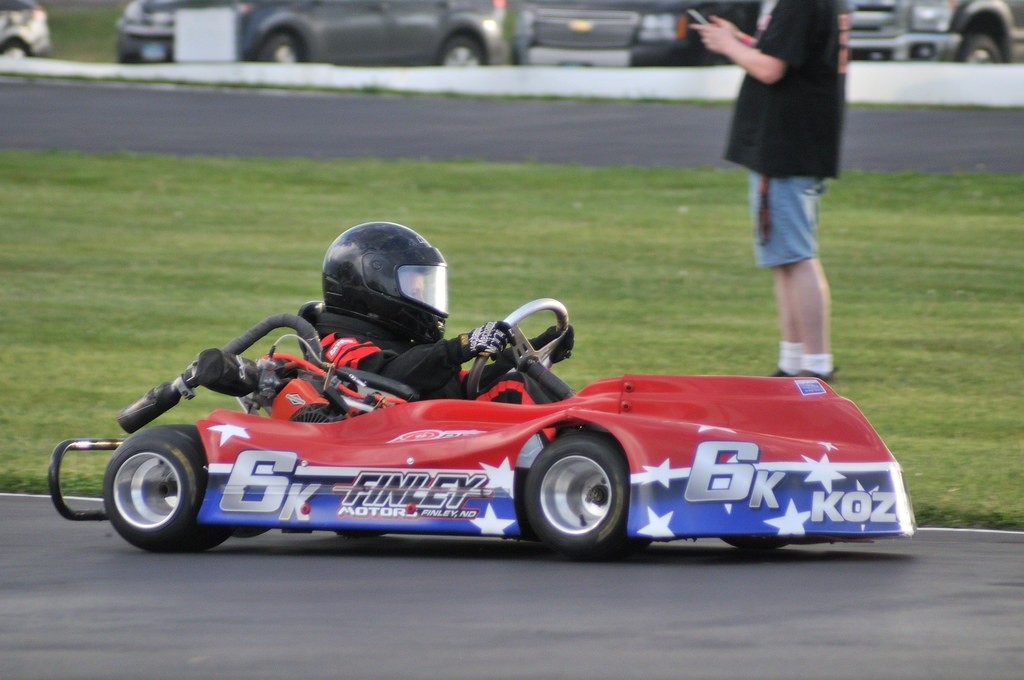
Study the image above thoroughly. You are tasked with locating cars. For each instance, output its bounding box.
[0,1,52,59]
[116,1,1023,66]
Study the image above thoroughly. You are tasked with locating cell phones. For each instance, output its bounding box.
[686,8,709,25]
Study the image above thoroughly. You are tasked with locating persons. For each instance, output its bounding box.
[688,0,850,383]
[313,222,575,405]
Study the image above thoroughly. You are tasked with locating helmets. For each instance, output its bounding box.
[322,221,449,343]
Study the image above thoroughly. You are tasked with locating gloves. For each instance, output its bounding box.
[528,324,574,363]
[458,320,516,363]
